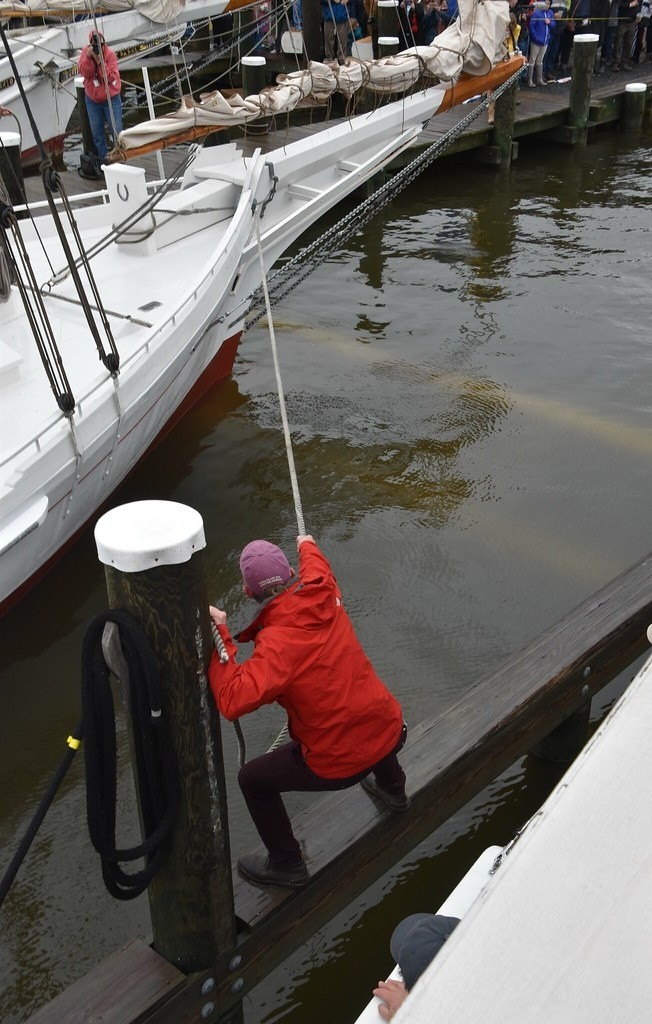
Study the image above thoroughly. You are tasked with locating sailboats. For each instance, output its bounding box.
[0,0,531,627]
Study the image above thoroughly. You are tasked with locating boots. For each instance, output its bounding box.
[528,65,537,87]
[535,62,547,86]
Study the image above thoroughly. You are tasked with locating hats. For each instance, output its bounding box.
[89,31,105,46]
[240,539,291,598]
[389,912,462,989]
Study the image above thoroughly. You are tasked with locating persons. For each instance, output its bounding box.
[372,912,462,1023]
[209,534,410,888]
[293,1,652,124]
[78,31,123,161]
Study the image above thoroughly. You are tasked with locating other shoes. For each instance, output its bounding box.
[362,772,410,812]
[612,61,620,72]
[238,850,312,889]
[623,62,632,70]
[599,59,606,72]
